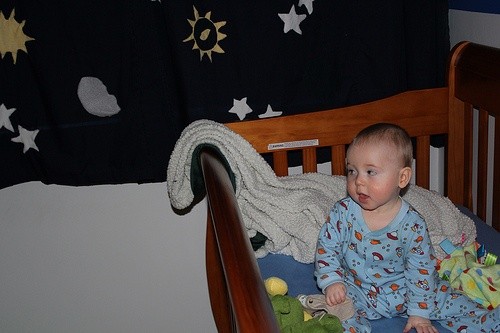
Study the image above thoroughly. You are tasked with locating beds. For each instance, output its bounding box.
[200,41,500,333]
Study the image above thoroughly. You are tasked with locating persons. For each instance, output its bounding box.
[313,123,500,333]
